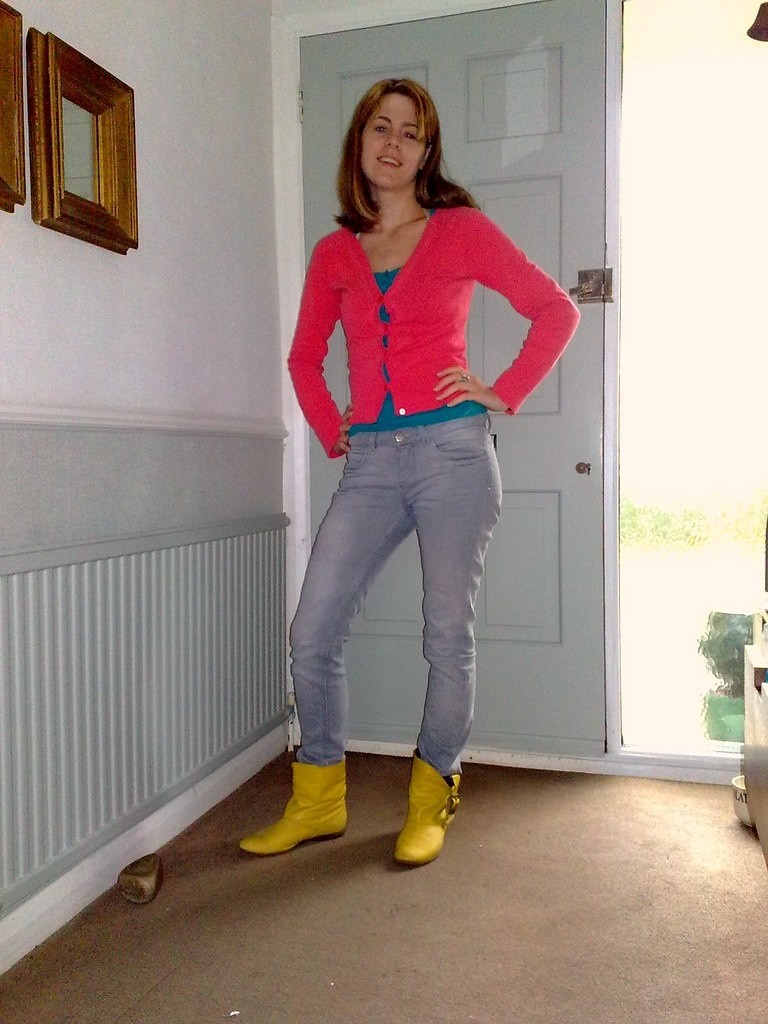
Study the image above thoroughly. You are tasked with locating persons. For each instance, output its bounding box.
[237,78,579,865]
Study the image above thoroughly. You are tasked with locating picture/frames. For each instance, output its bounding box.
[0,1,138,254]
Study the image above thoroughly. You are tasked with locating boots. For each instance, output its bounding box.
[394,748,461,865]
[240,755,348,856]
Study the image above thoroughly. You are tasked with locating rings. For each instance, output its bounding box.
[462,374,470,382]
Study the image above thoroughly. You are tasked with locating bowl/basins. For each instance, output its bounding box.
[732,776,755,827]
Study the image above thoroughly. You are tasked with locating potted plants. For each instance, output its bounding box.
[697,612,753,740]
